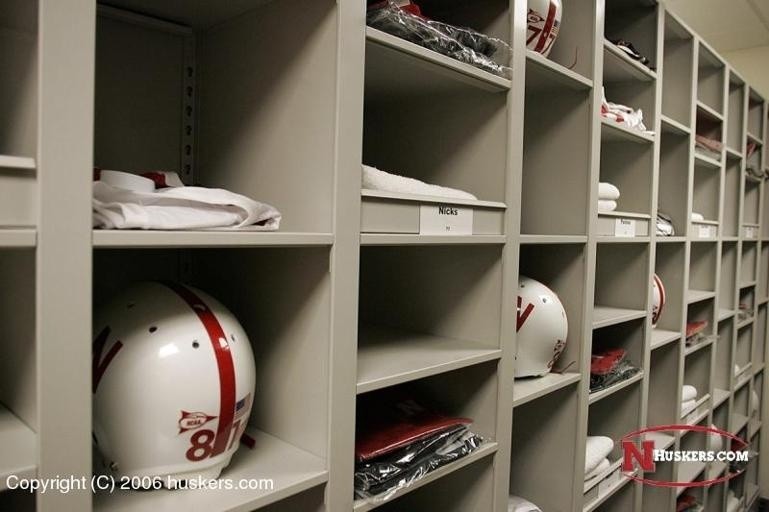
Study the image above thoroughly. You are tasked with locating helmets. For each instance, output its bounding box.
[92,278,256,489]
[527,0,562,59]
[515,276,569,380]
[653,273,666,329]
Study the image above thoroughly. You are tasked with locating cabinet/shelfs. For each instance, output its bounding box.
[0,0,769,512]
